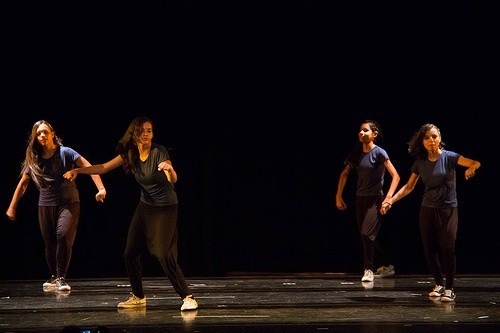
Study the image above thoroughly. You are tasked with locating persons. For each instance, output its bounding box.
[336,120,400,282]
[380,123,481,301]
[6,120,106,290]
[63,117,198,311]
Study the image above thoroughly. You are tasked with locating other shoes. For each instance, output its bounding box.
[42,274,57,287]
[361,268,374,282]
[440,287,456,302]
[373,265,395,278]
[428,284,446,297]
[55,276,72,290]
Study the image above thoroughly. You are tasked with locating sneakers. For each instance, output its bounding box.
[180,295,199,311]
[116,291,146,309]
